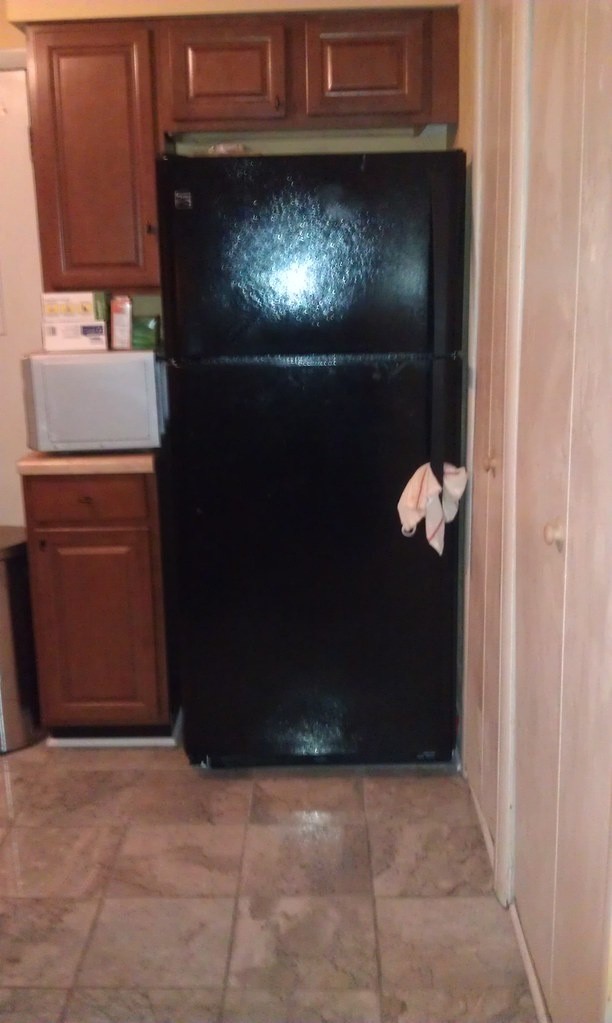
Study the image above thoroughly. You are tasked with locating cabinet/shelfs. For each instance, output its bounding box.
[20,9,459,292]
[25,474,169,731]
